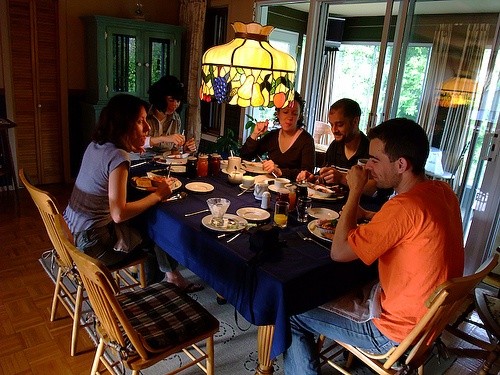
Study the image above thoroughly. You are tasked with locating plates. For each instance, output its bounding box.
[202,214,248,232]
[307,219,339,243]
[221,167,246,174]
[236,207,270,221]
[308,207,339,220]
[135,177,182,191]
[156,158,187,165]
[185,182,214,192]
[307,181,345,201]
[239,184,254,192]
[268,184,297,194]
[240,163,271,174]
[166,165,186,173]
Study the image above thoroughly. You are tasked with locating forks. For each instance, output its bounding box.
[296,231,331,252]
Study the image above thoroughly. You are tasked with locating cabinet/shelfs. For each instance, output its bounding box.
[69,14,184,174]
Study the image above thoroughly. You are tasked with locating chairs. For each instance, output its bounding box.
[18,168,220,375]
[316,246,500,375]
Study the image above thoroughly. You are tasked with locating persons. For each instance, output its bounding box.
[282,117,464,375]
[295,98,395,212]
[225,91,316,181]
[132,75,185,152]
[63,94,202,293]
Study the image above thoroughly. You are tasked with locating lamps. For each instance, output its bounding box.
[438,72,477,107]
[199,0,297,109]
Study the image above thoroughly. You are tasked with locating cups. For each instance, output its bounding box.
[296,196,312,223]
[226,157,241,173]
[275,177,290,191]
[206,198,230,228]
[274,201,289,228]
[357,159,371,179]
[242,176,254,187]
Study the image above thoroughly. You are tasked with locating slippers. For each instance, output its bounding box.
[182,281,205,293]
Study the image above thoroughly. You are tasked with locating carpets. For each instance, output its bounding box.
[39,258,500,375]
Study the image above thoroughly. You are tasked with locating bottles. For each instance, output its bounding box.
[185,153,222,179]
[260,191,271,209]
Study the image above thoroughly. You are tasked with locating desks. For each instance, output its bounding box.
[126,159,379,375]
